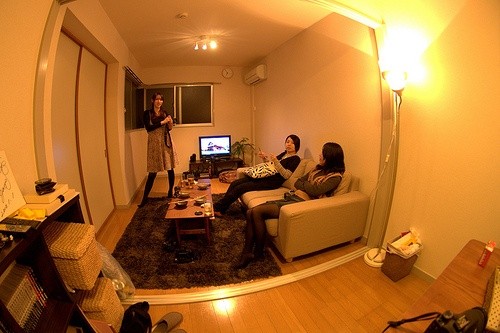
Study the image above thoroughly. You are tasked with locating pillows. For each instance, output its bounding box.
[242,160,280,178]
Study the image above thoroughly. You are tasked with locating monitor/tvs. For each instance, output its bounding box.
[199,135,232,160]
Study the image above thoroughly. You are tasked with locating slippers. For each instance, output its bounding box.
[150,312,182,333]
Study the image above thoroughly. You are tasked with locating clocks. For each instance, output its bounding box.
[222,66,234,79]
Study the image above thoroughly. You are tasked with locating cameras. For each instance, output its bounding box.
[423,306,488,333]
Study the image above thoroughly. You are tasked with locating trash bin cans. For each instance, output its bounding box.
[382,232,417,282]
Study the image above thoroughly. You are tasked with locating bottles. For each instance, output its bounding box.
[479,240,496,268]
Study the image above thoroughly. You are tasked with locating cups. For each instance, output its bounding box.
[204,203,212,215]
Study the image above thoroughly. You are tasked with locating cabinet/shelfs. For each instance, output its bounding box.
[0,191,105,333]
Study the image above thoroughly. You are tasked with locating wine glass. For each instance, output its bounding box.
[179,174,185,188]
[187,175,192,188]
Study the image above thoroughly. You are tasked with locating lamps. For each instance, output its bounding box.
[191,35,220,52]
[364,67,410,268]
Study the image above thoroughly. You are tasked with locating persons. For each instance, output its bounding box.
[231,142,345,269]
[213,135,301,217]
[137,92,177,208]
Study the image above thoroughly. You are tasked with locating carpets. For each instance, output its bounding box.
[110,192,285,291]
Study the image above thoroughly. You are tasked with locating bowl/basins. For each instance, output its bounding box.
[175,200,187,207]
[195,198,206,204]
[198,185,207,189]
[179,192,190,198]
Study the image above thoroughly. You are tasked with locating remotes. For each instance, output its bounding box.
[0,223,32,235]
[1,218,41,230]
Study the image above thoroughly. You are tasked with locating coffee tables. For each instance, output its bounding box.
[165,177,215,245]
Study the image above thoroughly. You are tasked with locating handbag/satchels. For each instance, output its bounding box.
[386,229,423,259]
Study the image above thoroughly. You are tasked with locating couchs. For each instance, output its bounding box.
[235,159,371,264]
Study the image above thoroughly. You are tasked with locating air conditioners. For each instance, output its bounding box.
[244,63,268,88]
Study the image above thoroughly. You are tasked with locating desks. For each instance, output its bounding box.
[387,237,500,333]
[183,156,244,179]
